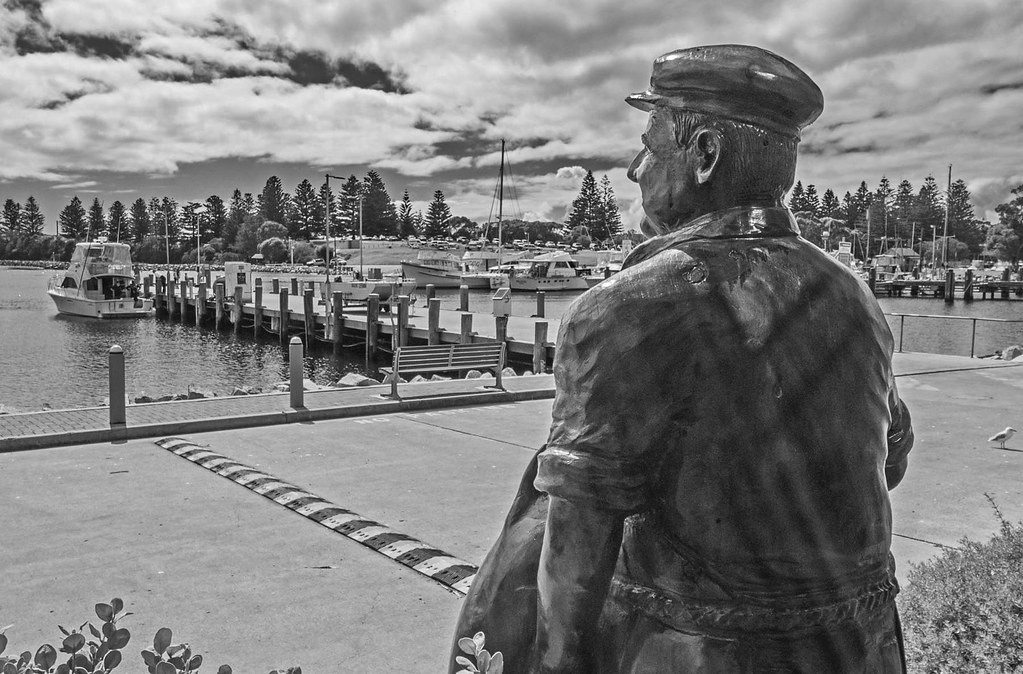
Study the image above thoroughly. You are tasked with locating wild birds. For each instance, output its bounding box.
[988,427,1018,449]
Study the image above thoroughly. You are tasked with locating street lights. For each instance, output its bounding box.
[324,172,347,280]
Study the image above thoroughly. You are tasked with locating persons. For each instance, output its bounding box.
[451,47,913,674]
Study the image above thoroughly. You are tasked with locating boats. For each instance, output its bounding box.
[400,251,463,287]
[512,251,591,292]
[46,240,156,322]
[318,279,412,302]
[463,250,512,290]
